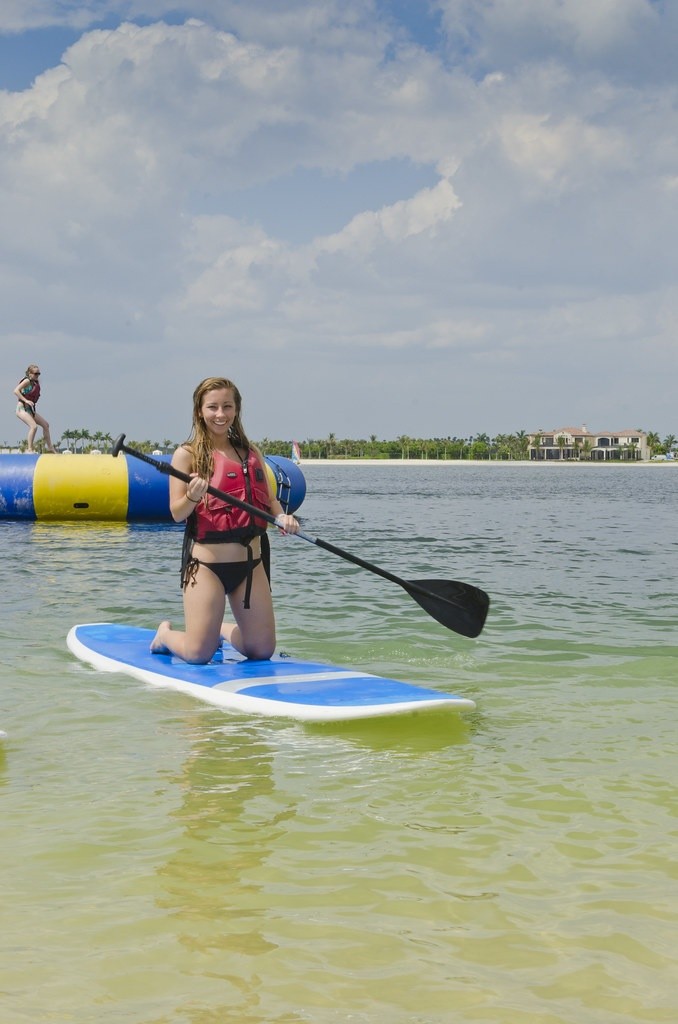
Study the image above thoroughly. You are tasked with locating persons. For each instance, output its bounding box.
[14,365,57,454]
[149,377,300,665]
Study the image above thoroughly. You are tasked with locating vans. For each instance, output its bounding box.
[652,455,666,460]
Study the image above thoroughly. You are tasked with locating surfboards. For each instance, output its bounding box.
[65,621,478,725]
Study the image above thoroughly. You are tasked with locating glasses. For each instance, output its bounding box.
[30,373,41,375]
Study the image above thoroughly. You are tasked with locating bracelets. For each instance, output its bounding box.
[186,494,199,502]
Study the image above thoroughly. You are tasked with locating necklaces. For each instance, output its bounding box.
[230,442,243,462]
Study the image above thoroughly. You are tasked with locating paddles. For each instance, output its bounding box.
[112,433,491,639]
